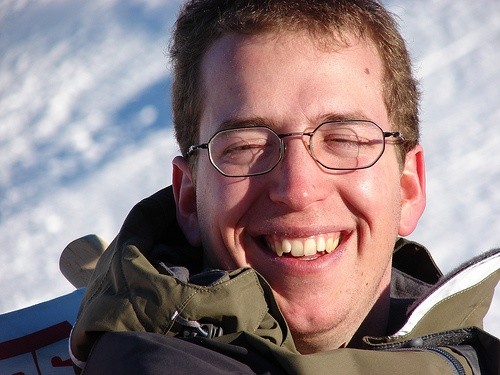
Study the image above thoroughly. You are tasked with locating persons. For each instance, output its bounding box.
[69,0,499,375]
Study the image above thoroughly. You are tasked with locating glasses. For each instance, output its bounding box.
[182,120,406,177]
